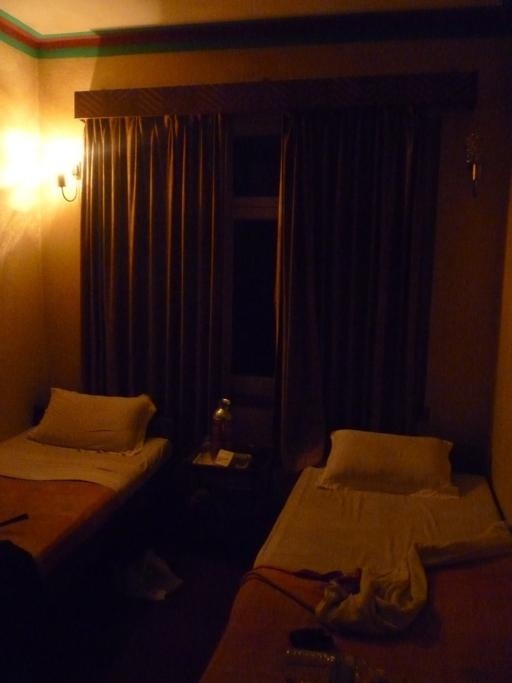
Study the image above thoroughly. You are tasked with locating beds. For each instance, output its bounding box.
[1,429,171,591]
[198,466,512,682]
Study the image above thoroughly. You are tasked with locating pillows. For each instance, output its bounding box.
[315,430,455,500]
[25,387,155,457]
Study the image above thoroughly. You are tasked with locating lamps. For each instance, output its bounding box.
[41,142,82,201]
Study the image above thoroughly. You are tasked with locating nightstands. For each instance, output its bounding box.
[186,446,277,567]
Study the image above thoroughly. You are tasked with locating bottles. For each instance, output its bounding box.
[212,397,235,459]
